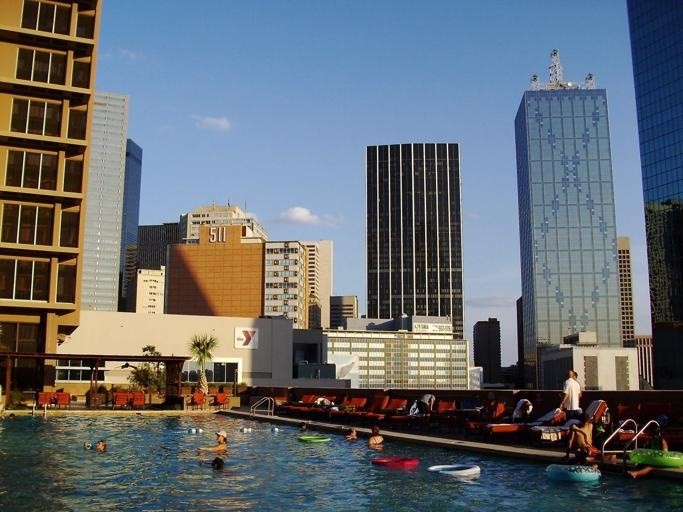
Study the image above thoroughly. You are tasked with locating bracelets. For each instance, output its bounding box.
[561,402,564,406]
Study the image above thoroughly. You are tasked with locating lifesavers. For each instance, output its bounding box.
[298,435,331,444]
[630,449,683,467]
[427,464,479,477]
[372,457,419,467]
[546,463,602,483]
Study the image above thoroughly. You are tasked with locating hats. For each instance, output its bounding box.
[215,431,226,438]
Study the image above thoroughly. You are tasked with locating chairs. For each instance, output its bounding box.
[274,395,683,449]
[36,392,231,410]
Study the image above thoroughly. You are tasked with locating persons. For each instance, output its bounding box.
[559,411,601,461]
[196,430,230,453]
[416,391,436,419]
[368,425,385,451]
[343,427,358,442]
[300,421,308,433]
[96,440,107,452]
[9,413,16,419]
[572,372,583,400]
[211,457,231,473]
[625,427,669,481]
[567,447,599,473]
[558,370,583,418]
[510,397,534,423]
